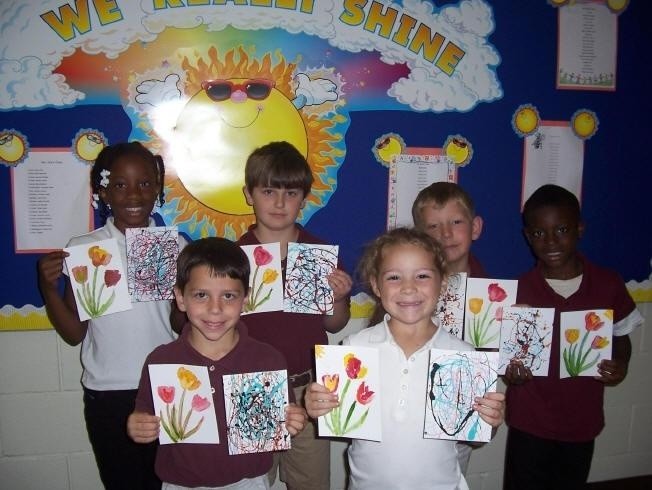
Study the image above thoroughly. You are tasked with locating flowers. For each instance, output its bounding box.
[155,368,209,443]
[241,247,278,311]
[468,282,503,349]
[562,312,607,377]
[321,353,373,436]
[71,247,122,320]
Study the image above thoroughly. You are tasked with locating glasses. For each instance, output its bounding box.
[202,79,276,102]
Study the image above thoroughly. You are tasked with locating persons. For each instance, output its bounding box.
[127,237,309,490]
[367,182,533,352]
[37,142,189,490]
[169,141,354,489]
[502,185,644,490]
[304,228,506,490]
